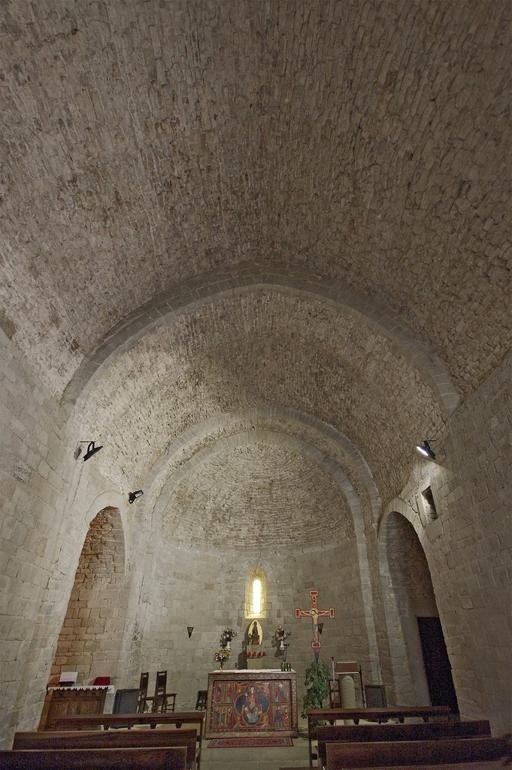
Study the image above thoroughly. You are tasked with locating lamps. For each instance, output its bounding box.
[80,440,103,462]
[129,490,143,504]
[415,438,439,460]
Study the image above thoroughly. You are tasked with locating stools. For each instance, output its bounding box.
[196,690,207,710]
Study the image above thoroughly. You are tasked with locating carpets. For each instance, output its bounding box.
[207,736,295,748]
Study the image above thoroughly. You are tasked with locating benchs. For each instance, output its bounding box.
[312,718,510,769]
[1,729,200,770]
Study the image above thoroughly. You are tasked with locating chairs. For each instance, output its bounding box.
[136,670,177,714]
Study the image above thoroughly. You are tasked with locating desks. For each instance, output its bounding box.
[36,686,110,731]
[306,706,450,767]
[52,711,205,756]
[203,672,301,740]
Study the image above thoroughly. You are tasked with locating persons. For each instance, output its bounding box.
[251,621,260,644]
[302,609,328,643]
